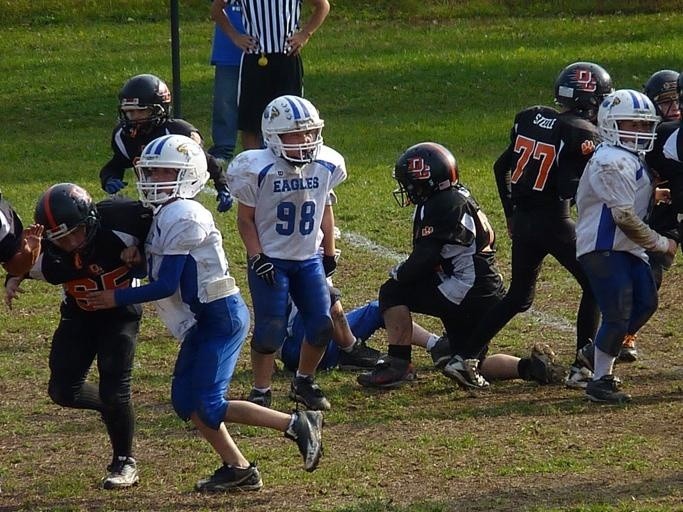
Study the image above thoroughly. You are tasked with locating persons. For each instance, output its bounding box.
[573,69,683,404]
[5,183,154,489]
[86,134,325,493]
[358,142,570,390]
[99,73,233,212]
[211,0,331,150]
[226,94,348,412]
[443,61,625,390]
[277,225,488,373]
[208,4,246,167]
[0,189,44,276]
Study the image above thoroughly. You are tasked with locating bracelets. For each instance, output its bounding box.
[302,30,311,38]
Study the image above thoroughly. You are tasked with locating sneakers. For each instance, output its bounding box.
[427,330,490,390]
[103,456,140,489]
[530,335,569,385]
[247,388,272,408]
[284,408,325,472]
[567,332,637,406]
[339,338,414,387]
[289,374,331,410]
[194,462,263,493]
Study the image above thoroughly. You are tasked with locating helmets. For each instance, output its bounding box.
[34,183,102,263]
[390,142,458,208]
[261,95,325,164]
[118,74,174,146]
[553,61,683,152]
[135,133,211,215]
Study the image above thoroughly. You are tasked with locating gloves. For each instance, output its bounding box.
[216,185,234,213]
[101,177,128,195]
[324,255,337,277]
[249,253,276,285]
[654,238,677,271]
